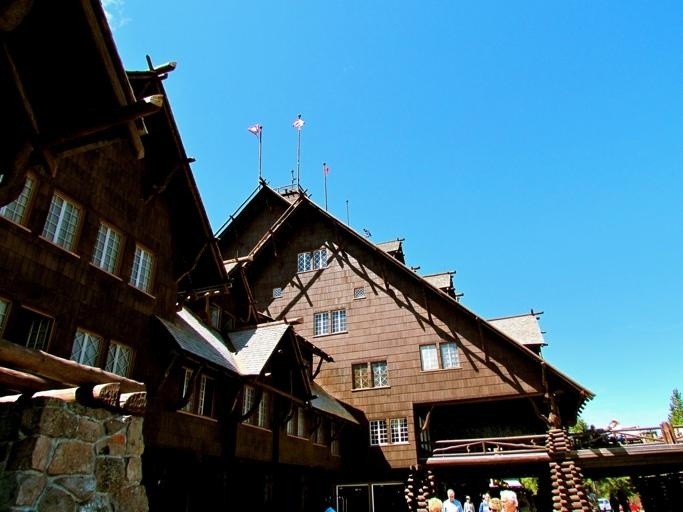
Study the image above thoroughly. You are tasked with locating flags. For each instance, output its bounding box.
[292,119,305,131]
[323,167,329,175]
[247,123,260,138]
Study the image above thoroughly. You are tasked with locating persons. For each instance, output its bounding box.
[609,492,620,511]
[606,419,625,444]
[486,497,502,512]
[616,489,632,511]
[463,495,475,512]
[428,489,442,512]
[442,487,463,511]
[478,492,491,511]
[588,424,608,448]
[499,489,519,512]
